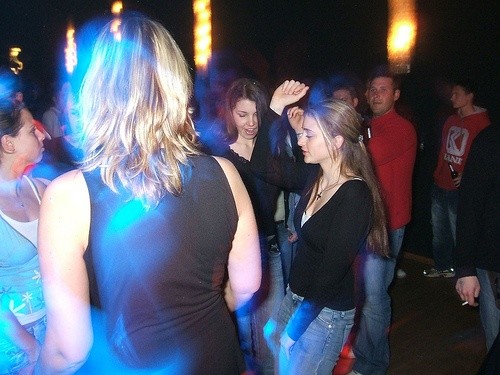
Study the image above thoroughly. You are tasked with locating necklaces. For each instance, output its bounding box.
[315,177,343,201]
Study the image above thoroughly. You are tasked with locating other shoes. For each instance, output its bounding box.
[340,344,356,359]
[347,370,362,375]
[395,267,407,279]
[422,266,456,278]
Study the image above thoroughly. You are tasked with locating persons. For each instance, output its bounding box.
[450,118,500,374]
[336,70,418,373]
[364,70,383,105]
[271,185,306,296]
[15,76,76,156]
[247,79,390,374]
[419,82,489,282]
[33,13,264,375]
[204,77,286,374]
[0,96,46,375]
[326,82,362,118]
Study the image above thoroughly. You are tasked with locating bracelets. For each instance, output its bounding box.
[296,133,302,136]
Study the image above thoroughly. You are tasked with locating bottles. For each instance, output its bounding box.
[366,125,372,140]
[449,161,462,190]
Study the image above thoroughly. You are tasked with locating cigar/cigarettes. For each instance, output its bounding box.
[460,300,469,307]
